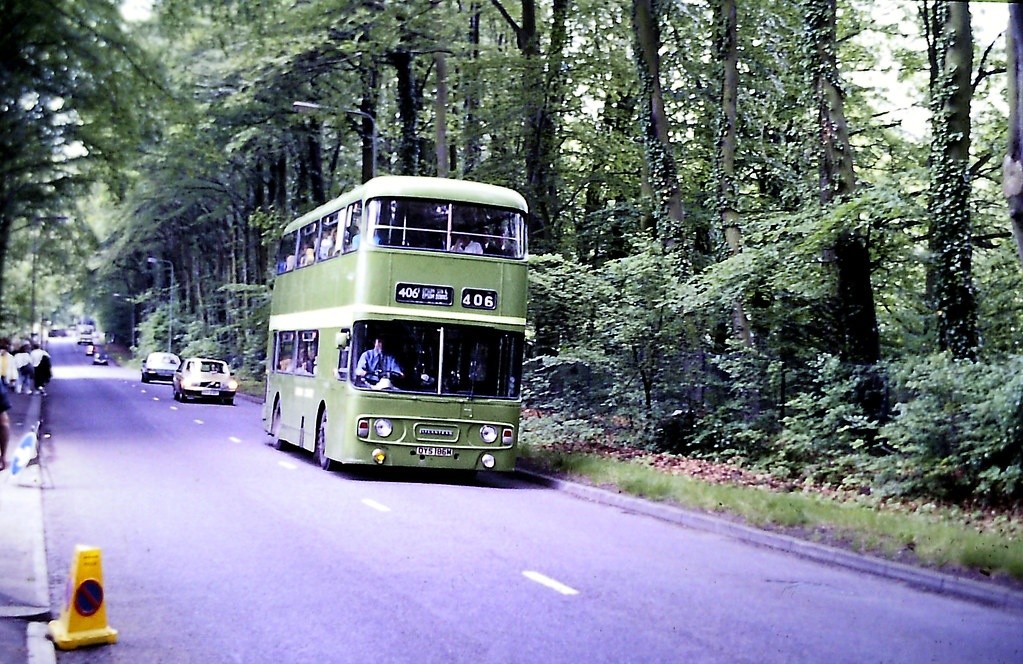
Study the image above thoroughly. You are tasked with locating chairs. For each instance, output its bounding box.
[278,231,361,273]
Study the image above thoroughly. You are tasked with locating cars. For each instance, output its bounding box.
[172,356,239,405]
[140,352,182,383]
[48,322,109,365]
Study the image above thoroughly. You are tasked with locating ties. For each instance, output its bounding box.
[378,354,383,371]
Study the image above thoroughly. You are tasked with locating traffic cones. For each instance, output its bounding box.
[46,546,119,650]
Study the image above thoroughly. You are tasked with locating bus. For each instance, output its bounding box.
[262,175,530,474]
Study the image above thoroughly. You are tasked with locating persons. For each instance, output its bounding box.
[278,217,517,391]
[0,337,52,469]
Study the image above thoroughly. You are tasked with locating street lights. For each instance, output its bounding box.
[292,98,378,178]
[145,256,175,353]
[29,216,68,341]
[113,293,135,360]
